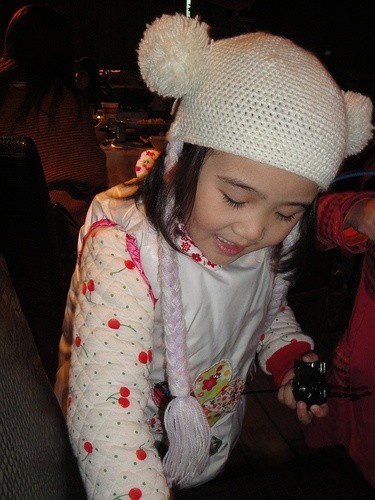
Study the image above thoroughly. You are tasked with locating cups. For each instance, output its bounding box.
[102,102,119,121]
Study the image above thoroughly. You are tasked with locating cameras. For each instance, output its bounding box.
[291,361,327,411]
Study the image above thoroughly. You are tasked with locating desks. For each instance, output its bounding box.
[109,113,172,141]
[99,138,160,187]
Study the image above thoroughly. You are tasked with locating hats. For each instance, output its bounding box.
[136,12,374,193]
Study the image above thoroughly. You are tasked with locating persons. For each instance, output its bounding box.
[0,5,108,223]
[73,56,114,115]
[304,152,375,488]
[50,14,375,500]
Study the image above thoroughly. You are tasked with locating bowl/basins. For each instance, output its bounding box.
[128,120,161,136]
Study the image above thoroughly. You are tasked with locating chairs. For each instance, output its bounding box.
[0,134,102,261]
